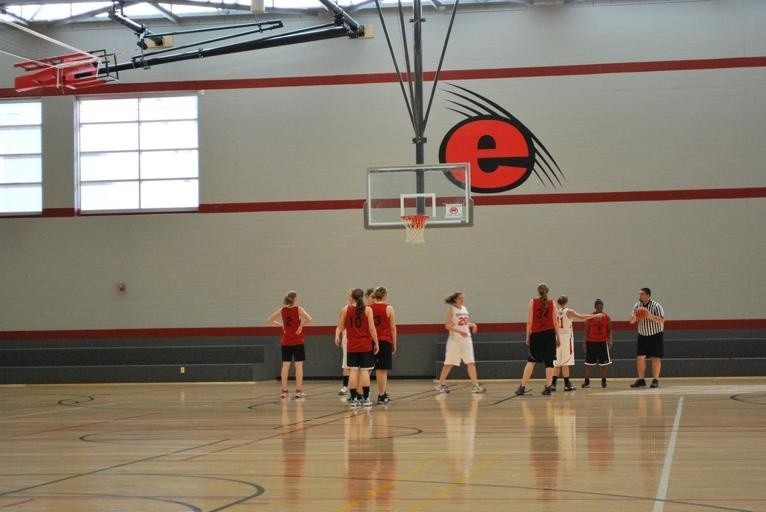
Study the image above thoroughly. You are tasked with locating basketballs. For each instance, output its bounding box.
[635,307,647,321]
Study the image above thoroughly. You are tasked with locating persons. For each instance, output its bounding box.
[333,286,397,406]
[550,294,605,391]
[434,291,487,395]
[580,299,613,388]
[515,283,561,395]
[266,290,313,398]
[629,287,666,388]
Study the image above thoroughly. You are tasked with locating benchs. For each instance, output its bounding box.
[435,338,766,379]
[0,345,264,384]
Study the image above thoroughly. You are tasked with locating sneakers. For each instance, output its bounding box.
[339,386,391,406]
[630,378,646,388]
[434,383,450,394]
[564,383,576,391]
[601,377,607,388]
[582,382,591,388]
[515,384,526,395]
[295,388,306,398]
[471,384,488,393]
[541,384,556,396]
[650,378,659,388]
[280,388,289,398]
